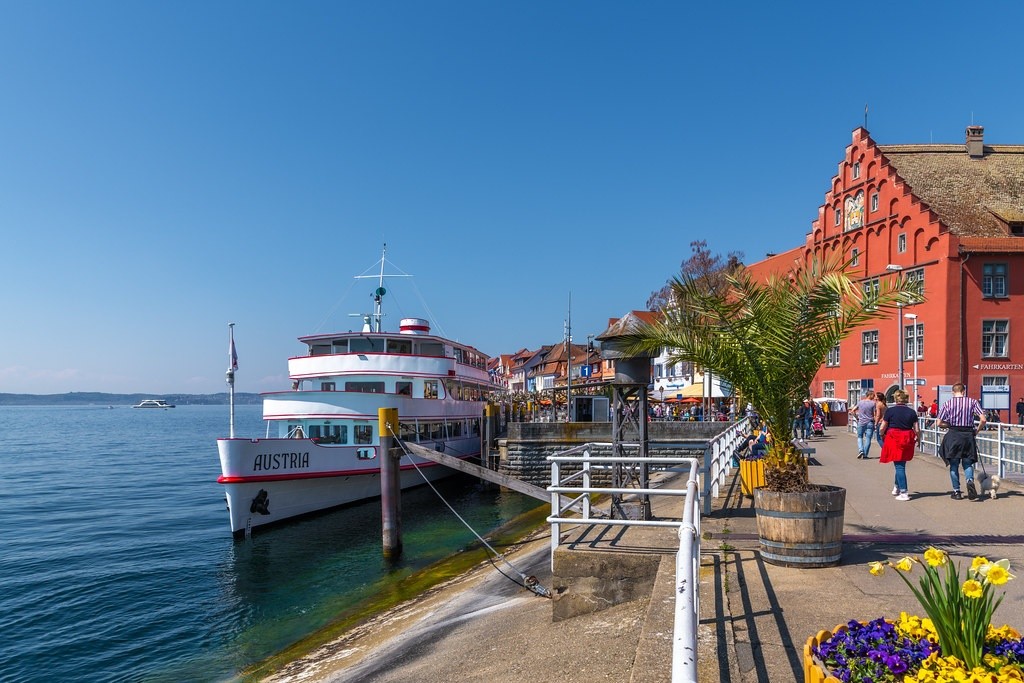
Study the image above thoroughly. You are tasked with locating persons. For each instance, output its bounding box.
[981,410,1005,431]
[792,396,830,442]
[875,392,888,458]
[879,391,921,500]
[609,400,752,422]
[1016,398,1024,425]
[937,383,986,501]
[851,391,876,459]
[736,415,776,461]
[917,395,938,428]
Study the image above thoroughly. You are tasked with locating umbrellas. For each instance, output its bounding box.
[664,397,700,405]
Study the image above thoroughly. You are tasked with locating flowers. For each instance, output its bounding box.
[812,545,1024,683]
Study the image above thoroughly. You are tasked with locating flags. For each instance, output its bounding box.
[229,326,238,367]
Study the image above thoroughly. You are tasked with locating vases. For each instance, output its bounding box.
[802,619,892,683]
[738,457,808,496]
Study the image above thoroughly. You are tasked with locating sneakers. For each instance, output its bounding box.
[895,493,911,501]
[966,480,977,500]
[892,486,900,496]
[951,490,962,500]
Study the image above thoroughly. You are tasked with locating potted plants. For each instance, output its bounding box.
[614,240,933,571]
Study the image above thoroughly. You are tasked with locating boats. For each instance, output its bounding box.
[133,399,176,408]
[217,239,504,540]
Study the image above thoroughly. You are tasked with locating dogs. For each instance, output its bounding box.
[976,470,1001,499]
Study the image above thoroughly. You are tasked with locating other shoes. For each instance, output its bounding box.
[791,437,797,442]
[799,438,804,442]
[863,456,871,460]
[856,451,864,459]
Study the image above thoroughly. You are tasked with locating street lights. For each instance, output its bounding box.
[586,333,595,396]
[885,264,904,392]
[904,313,918,415]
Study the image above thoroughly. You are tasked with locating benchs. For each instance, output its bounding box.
[794,441,816,463]
